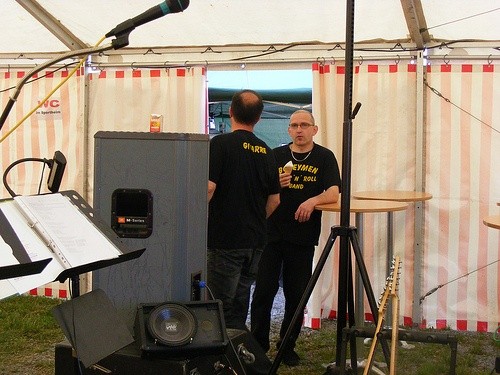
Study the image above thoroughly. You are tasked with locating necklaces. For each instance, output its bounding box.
[291,146,311,161]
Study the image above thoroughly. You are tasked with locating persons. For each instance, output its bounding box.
[250,109,341,364]
[207,89,280,375]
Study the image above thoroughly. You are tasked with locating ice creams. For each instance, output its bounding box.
[284,161,293,174]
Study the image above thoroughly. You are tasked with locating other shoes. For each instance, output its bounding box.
[276,341,299,366]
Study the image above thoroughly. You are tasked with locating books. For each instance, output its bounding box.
[0,193,123,299]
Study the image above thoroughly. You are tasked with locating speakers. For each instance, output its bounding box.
[56,130,273,375]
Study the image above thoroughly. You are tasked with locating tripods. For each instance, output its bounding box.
[268,0,393,375]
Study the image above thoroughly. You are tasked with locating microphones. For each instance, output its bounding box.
[104,0,190,38]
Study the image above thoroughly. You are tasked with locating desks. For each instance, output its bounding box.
[315,190,433,369]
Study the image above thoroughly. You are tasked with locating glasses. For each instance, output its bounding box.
[290,122,314,129]
